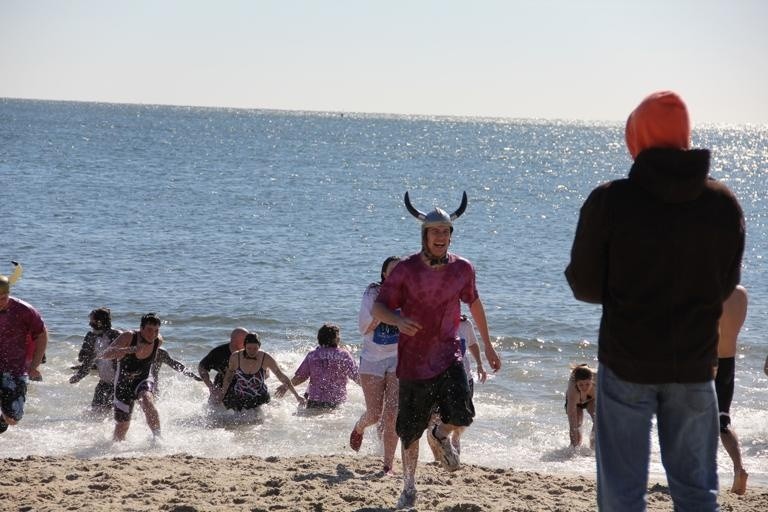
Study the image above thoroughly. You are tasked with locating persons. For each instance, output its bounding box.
[69,308,202,440]
[369,206,503,509]
[351,256,404,475]
[565,89,747,509]
[198,327,307,413]
[715,285,747,500]
[275,324,362,410]
[429,314,488,461]
[0,275,48,432]
[565,364,598,450]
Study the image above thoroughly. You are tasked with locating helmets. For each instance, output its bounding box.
[404,190,467,266]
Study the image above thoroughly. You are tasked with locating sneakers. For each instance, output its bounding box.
[432,425,459,472]
[396,491,416,511]
[350,421,364,453]
